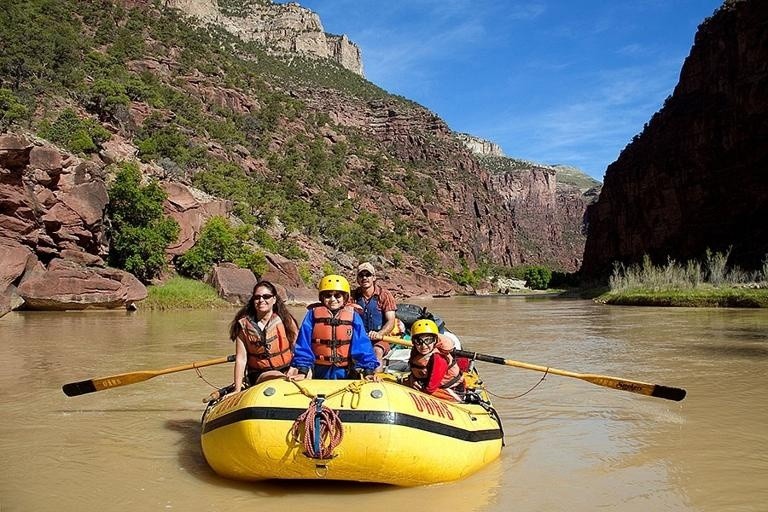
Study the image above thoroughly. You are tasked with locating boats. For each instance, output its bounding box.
[199,303,504,487]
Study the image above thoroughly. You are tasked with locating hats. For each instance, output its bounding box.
[358,262,375,274]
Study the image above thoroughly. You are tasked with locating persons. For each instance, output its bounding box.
[288,275,384,382]
[349,261,398,373]
[217,281,300,402]
[407,319,465,403]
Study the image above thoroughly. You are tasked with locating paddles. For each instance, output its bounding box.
[377,334,687,401]
[62,354,237,397]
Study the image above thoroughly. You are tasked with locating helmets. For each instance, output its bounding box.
[411,319,439,336]
[319,275,350,293]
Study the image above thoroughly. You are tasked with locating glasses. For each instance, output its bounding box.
[359,273,371,277]
[413,337,434,345]
[322,293,342,298]
[253,294,274,299]
